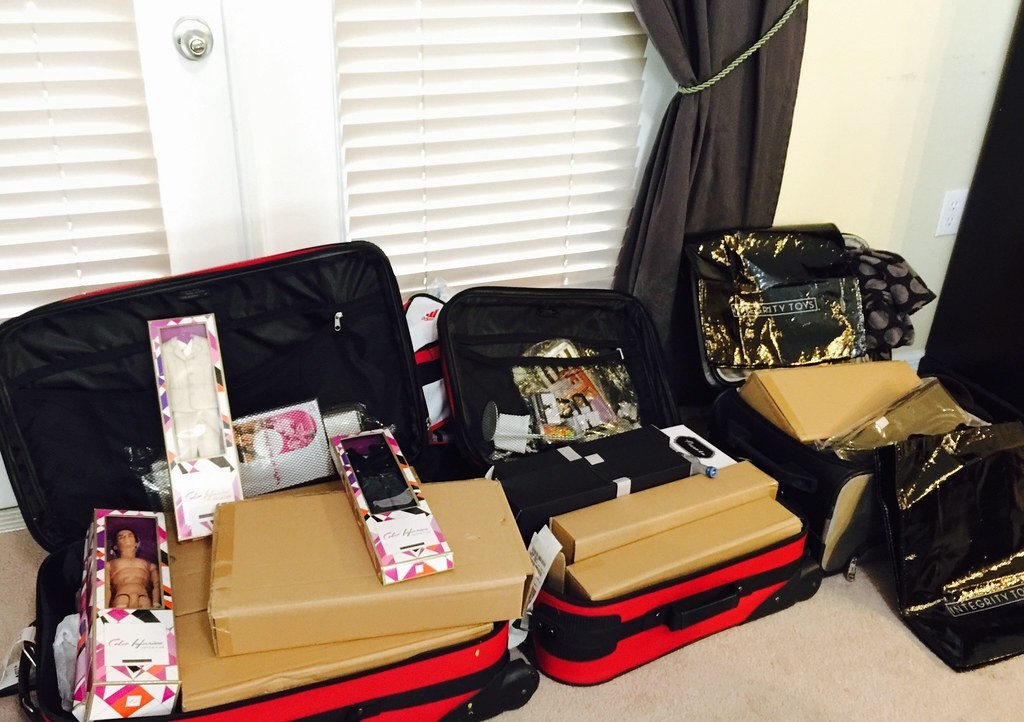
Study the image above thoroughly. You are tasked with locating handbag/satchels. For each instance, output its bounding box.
[862,423,1024,672]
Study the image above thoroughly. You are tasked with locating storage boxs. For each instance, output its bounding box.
[164,480,534,712]
[491,424,803,601]
[73,509,180,722]
[330,428,454,586]
[739,360,976,463]
[148,313,244,541]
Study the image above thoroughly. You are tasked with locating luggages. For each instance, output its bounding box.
[0,224,995,722]
